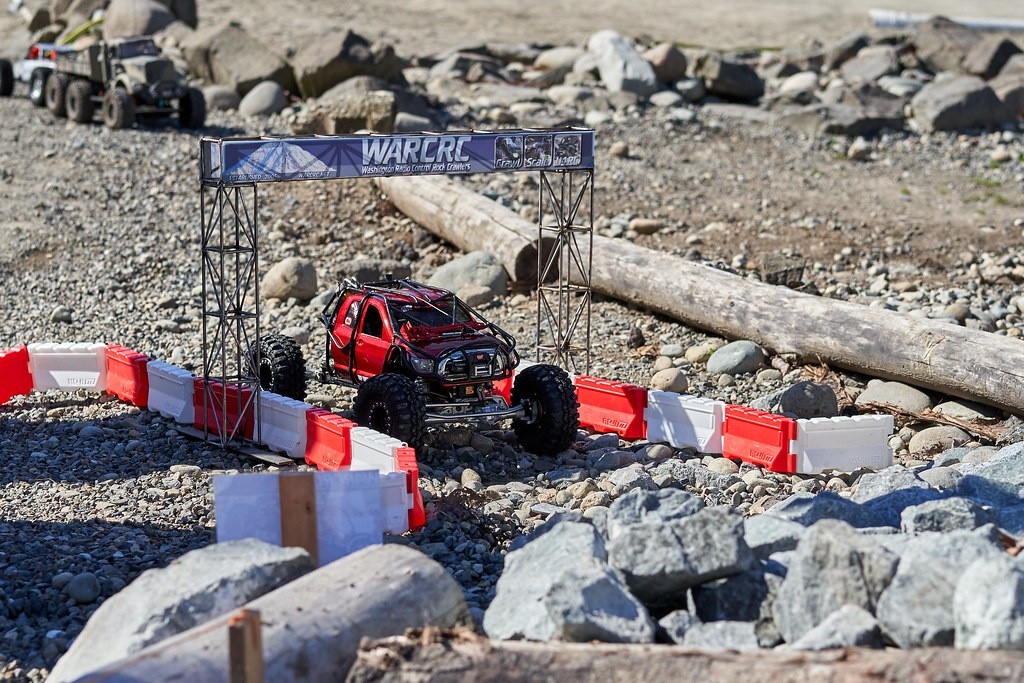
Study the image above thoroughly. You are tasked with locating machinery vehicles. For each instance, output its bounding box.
[11,9,107,82]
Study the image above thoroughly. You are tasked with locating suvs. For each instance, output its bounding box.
[243,275,583,464]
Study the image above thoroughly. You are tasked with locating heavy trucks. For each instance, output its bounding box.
[29,36,207,130]
